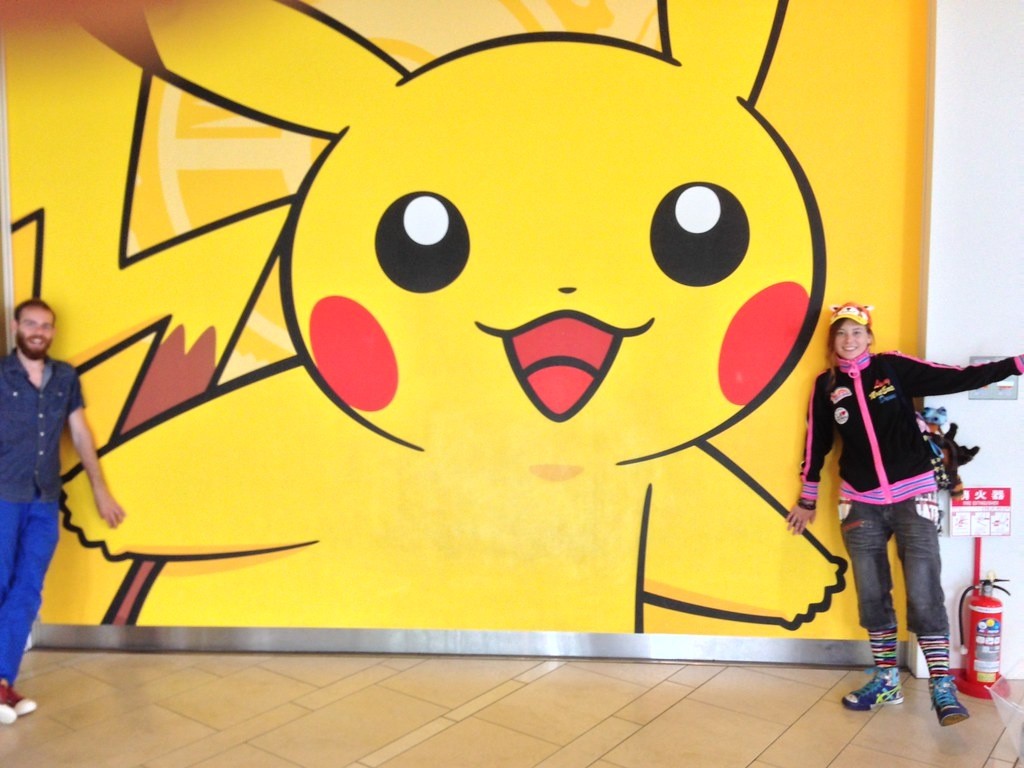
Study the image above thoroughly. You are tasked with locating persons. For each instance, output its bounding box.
[0,298,127,725]
[788,303,1024,727]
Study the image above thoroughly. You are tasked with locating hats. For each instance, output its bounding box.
[828,301,874,329]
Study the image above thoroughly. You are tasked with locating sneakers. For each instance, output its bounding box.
[843,668,904,710]
[0,683,37,725]
[928,676,970,727]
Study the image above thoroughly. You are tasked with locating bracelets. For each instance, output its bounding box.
[797,502,816,511]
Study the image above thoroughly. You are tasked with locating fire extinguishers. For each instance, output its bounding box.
[957,571,1012,689]
